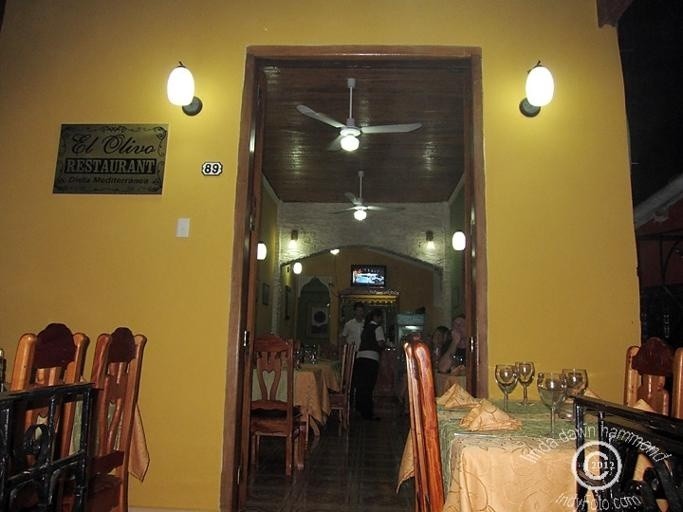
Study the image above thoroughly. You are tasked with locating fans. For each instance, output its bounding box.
[296,78,423,152]
[328,170,405,215]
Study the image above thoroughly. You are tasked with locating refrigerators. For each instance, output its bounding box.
[395,313,424,346]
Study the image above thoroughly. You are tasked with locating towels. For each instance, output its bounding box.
[632,399,658,414]
[460,399,522,430]
[436,384,478,408]
[565,387,600,403]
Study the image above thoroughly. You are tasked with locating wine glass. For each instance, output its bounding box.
[561,368,587,422]
[295,343,323,371]
[515,361,537,407]
[495,364,519,414]
[537,372,567,439]
[449,351,466,367]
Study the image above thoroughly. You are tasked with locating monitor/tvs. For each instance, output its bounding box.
[351,264,387,286]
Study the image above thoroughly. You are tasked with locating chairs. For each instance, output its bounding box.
[63,327,147,512]
[8,323,89,512]
[404,340,444,512]
[250,332,357,482]
[623,337,683,420]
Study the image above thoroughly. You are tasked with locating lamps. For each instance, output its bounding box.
[426,230,433,241]
[519,60,554,117]
[451,230,466,251]
[354,207,367,221]
[291,230,299,240]
[339,128,362,152]
[168,60,202,117]
[256,241,267,261]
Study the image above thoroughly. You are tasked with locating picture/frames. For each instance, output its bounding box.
[286,285,292,319]
[263,282,269,305]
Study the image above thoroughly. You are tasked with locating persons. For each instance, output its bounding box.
[430,326,451,363]
[340,300,365,405]
[438,313,466,375]
[352,307,386,419]
